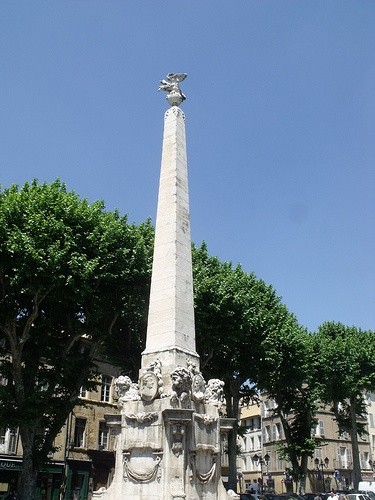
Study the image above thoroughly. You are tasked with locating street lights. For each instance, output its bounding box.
[315,456,329,494]
[252,453,270,495]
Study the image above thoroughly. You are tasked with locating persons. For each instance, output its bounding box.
[238,486,375,500]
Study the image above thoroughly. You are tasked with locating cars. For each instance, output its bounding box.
[240,490,375,500]
